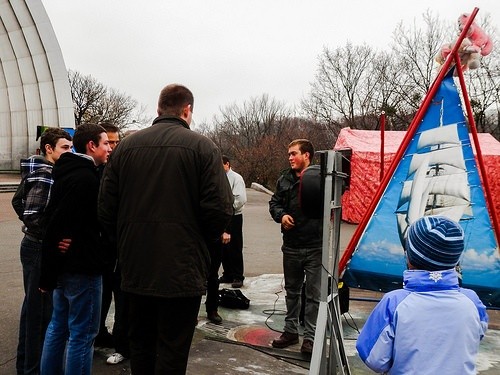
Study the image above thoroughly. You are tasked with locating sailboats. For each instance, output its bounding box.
[394,100,475,284]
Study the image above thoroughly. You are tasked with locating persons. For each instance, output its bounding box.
[94,83,234,375]
[195,155,247,327]
[356,216,489,375]
[269,139,347,355]
[40,123,111,375]
[97,123,131,365]
[12,128,74,375]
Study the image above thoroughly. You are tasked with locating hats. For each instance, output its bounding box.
[406,216,465,270]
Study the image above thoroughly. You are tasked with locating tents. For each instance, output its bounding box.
[333,126,500,229]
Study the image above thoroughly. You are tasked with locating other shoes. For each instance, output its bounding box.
[106,352,122,364]
[232,279,243,287]
[206,312,222,323]
[220,277,231,283]
[272,332,299,348]
[301,339,313,355]
